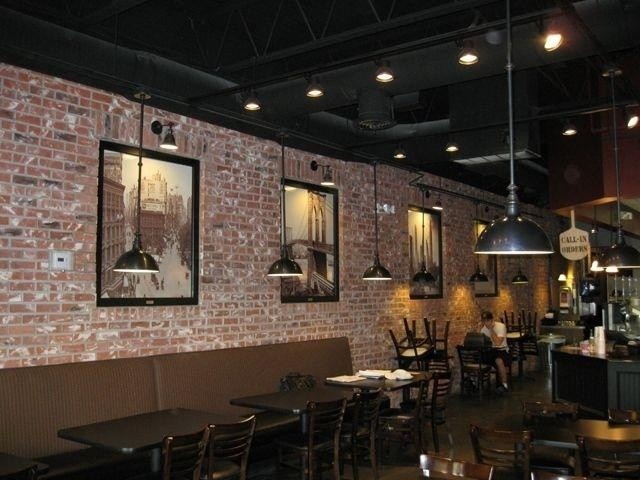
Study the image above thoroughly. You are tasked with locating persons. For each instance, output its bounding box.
[481,311,510,397]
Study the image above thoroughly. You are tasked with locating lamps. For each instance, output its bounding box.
[423,188,444,211]
[468,198,492,283]
[146,117,180,151]
[309,158,339,188]
[590,63,639,272]
[412,185,436,285]
[262,131,306,278]
[111,98,165,275]
[241,13,564,112]
[470,1,556,258]
[358,160,393,285]
[509,254,532,287]
[392,102,639,163]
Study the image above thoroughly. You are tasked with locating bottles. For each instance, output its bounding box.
[589,329,595,354]
[610,274,638,298]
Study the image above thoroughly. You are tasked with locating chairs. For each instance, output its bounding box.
[421,402,640,480]
[1,316,456,480]
[456,309,538,403]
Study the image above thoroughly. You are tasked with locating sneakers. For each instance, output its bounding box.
[495,384,509,396]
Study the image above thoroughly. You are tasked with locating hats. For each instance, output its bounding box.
[480,311,494,321]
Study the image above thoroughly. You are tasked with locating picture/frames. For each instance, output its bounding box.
[472,217,500,298]
[408,202,444,301]
[89,137,202,308]
[277,174,342,304]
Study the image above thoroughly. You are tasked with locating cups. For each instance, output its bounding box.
[580,341,589,353]
[594,324,606,356]
